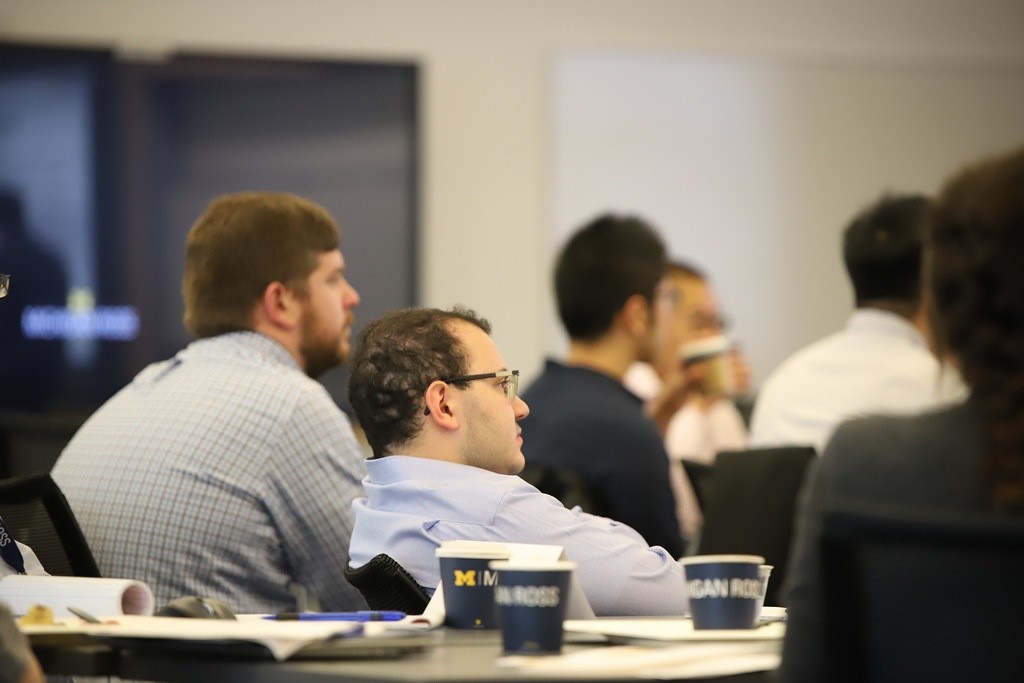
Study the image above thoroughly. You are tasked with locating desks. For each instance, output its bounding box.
[18,606,787,683]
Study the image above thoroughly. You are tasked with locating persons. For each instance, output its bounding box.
[348,306,689,619]
[513,216,686,555]
[745,191,971,457]
[625,259,745,554]
[775,148,1024,683]
[30,194,370,615]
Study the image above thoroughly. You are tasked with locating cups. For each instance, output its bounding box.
[433,542,513,629]
[488,560,578,658]
[679,553,774,632]
[675,335,749,411]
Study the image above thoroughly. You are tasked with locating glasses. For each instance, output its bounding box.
[423,370,519,415]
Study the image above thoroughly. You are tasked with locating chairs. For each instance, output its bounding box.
[681,444,819,607]
[0,472,128,683]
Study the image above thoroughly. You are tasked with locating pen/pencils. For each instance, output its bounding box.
[64,606,99,624]
[261,610,407,622]
